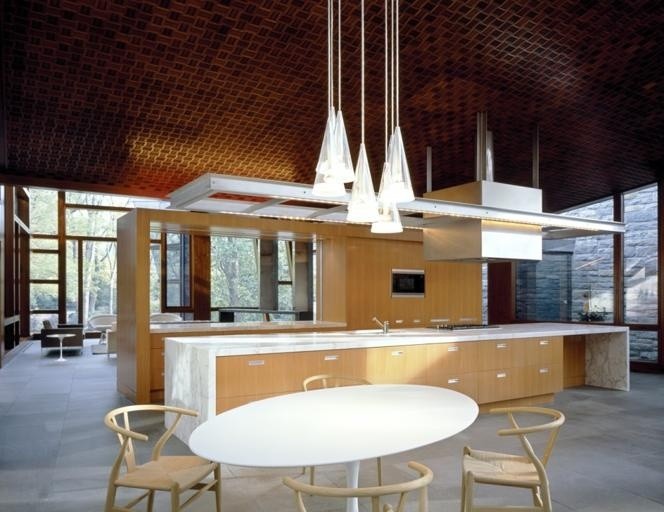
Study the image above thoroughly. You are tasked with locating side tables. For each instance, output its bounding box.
[45,333,76,361]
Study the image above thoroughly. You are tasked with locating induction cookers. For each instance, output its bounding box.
[427,323,500,331]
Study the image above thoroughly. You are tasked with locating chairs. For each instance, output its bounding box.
[151,313,183,323]
[104,402,223,512]
[283,373,434,511]
[41,320,85,356]
[460,405,566,512]
[87,314,119,359]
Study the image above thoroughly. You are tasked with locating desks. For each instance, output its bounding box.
[189,383,481,511]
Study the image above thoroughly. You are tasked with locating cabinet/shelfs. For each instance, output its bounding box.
[429,337,565,405]
[216,343,429,413]
[150,334,168,405]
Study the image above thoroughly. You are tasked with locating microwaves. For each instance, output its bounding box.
[390,269,425,298]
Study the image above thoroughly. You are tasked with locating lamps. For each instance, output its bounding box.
[311,0,419,238]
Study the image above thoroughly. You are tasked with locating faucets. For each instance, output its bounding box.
[372,316,385,330]
